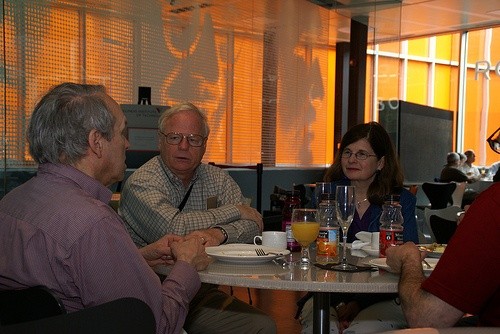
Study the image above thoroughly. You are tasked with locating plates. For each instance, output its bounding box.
[417,243,447,258]
[370,258,440,276]
[361,246,380,257]
[205,243,290,264]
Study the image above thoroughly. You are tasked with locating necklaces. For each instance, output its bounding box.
[357,199,367,207]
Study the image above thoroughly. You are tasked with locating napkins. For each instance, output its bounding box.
[351,231,379,251]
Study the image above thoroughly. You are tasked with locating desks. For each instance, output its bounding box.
[155,244,439,334]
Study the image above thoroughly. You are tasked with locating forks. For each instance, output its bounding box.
[254,248,281,256]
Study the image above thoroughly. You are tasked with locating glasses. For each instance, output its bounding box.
[487,127,500,154]
[160,129,207,147]
[341,149,378,160]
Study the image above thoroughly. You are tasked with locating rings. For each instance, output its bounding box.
[202,237,204,240]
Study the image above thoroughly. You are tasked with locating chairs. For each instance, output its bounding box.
[422,181,467,244]
[0,285,157,334]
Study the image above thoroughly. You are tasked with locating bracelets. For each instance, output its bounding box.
[222,229,227,236]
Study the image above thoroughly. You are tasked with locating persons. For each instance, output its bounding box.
[457,150,480,178]
[0,82,209,334]
[384,181,500,334]
[440,152,480,208]
[489,161,500,175]
[117,103,277,334]
[300,122,419,334]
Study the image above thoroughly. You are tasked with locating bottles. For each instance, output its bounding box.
[379,194,404,258]
[316,193,339,265]
[281,191,304,253]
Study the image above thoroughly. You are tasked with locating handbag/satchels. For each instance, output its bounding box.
[332,290,400,323]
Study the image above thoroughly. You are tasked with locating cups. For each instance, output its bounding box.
[315,182,334,197]
[253,231,287,250]
[466,168,472,177]
[480,166,486,178]
[370,232,380,250]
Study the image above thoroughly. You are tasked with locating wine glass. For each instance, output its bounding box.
[291,208,321,266]
[331,185,358,270]
